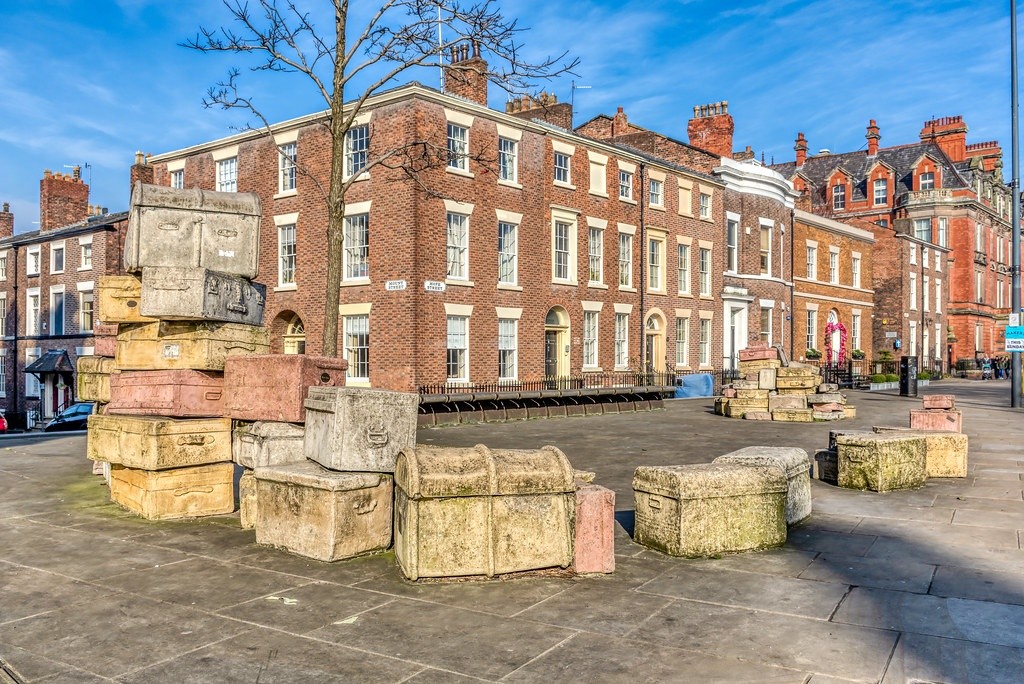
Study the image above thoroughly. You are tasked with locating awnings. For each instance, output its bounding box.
[25,349,74,382]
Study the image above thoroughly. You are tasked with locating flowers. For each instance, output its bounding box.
[825,322,848,369]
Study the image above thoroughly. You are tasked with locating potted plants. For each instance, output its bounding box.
[916,372,931,387]
[870,374,886,390]
[805,349,822,360]
[886,374,901,390]
[852,349,866,360]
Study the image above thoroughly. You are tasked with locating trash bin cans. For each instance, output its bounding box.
[900,356,918,397]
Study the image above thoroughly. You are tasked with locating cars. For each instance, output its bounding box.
[0,413,8,434]
[44,402,94,430]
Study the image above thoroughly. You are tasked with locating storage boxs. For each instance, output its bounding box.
[638,348,969,558]
[109,463,236,520]
[223,354,615,582]
[109,370,226,418]
[77,273,160,404]
[123,179,263,280]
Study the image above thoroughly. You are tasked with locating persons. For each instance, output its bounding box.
[980,353,991,379]
[997,357,1007,379]
[991,356,999,378]
[1004,356,1010,379]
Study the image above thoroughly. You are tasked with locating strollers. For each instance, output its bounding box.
[983,364,992,380]
